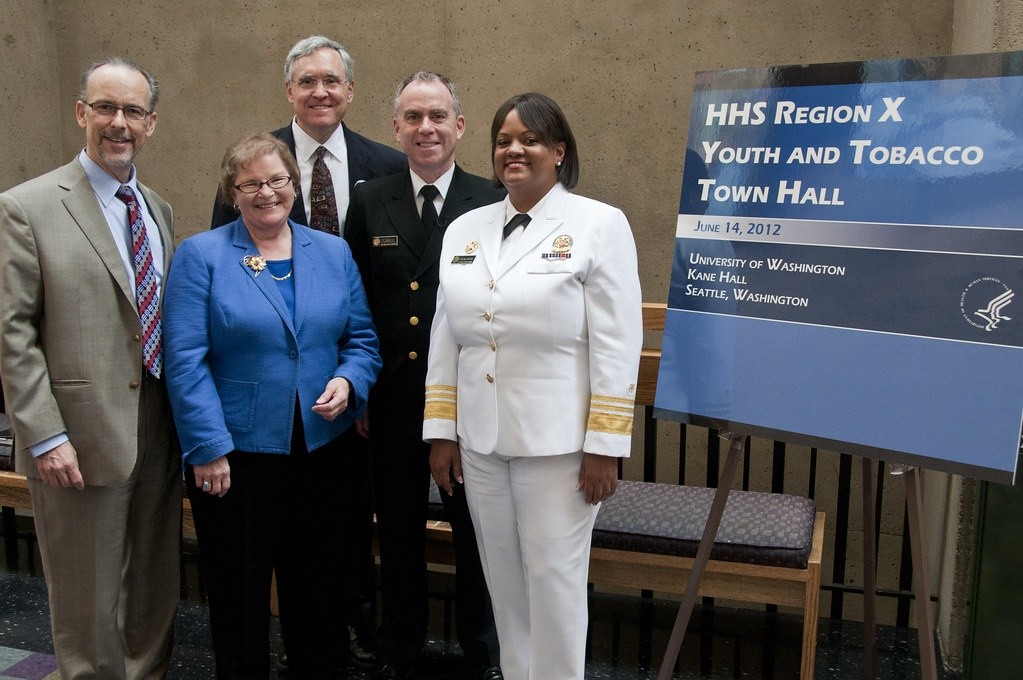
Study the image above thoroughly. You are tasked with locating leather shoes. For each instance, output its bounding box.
[277,644,289,671]
[372,664,415,680]
[480,665,504,680]
[349,639,380,665]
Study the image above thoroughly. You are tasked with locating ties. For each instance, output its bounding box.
[115,187,163,380]
[309,146,339,236]
[421,185,439,238]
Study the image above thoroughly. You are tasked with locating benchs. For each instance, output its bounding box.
[426,303,826,680]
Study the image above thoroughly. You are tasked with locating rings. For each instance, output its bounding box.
[203,482,212,489]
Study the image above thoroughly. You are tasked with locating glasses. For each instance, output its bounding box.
[290,77,347,88]
[233,176,292,194]
[82,99,153,121]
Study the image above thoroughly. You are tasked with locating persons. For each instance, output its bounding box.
[0,54,178,680]
[162,132,384,680]
[208,36,412,678]
[423,92,644,680]
[345,67,516,680]
